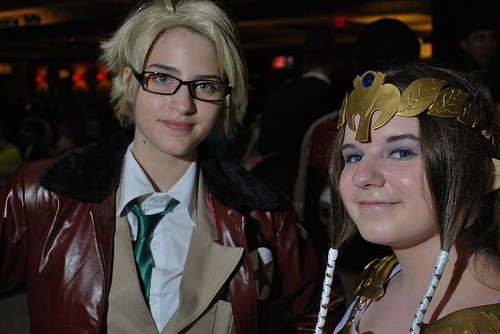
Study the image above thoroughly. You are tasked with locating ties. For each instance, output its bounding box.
[124,198,180,305]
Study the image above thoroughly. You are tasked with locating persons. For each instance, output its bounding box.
[0,0,348,334]
[252,19,500,334]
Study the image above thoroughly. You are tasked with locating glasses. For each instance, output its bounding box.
[128,61,233,102]
